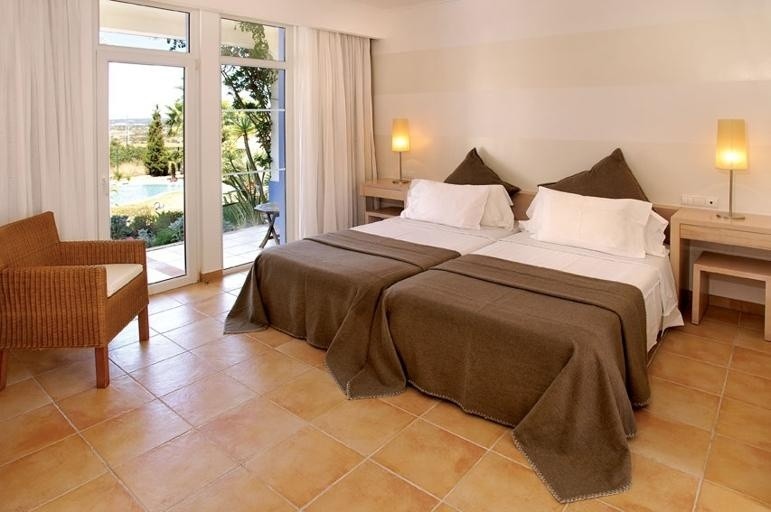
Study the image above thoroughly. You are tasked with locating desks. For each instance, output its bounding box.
[254,202,280,248]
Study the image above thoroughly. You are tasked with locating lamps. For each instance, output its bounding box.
[391,117,409,184]
[715,119,747,220]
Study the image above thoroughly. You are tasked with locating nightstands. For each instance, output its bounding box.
[359,180,411,224]
[669,208,771,309]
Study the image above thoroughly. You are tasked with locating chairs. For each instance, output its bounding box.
[0,211,149,388]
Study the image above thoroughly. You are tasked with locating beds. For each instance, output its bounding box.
[221,216,521,397]
[346,228,685,503]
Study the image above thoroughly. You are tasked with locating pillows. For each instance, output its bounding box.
[400,148,669,258]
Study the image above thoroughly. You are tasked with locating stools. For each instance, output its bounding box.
[365,206,402,225]
[692,252,771,342]
